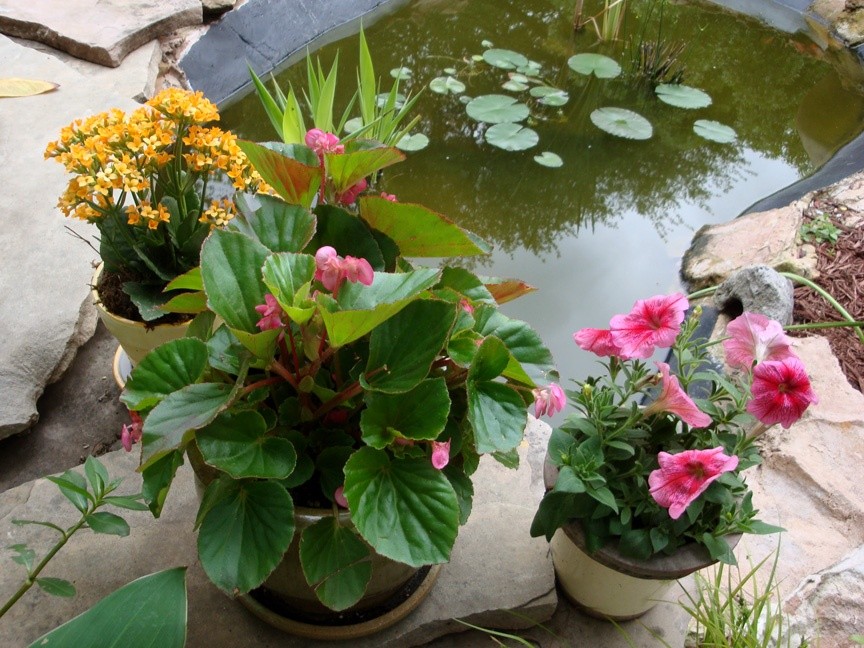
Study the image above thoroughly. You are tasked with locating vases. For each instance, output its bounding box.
[87,262,220,404]
[182,444,445,641]
[542,428,749,621]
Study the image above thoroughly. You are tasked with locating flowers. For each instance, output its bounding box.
[534,294,810,544]
[124,229,557,612]
[261,28,428,209]
[42,90,257,282]
